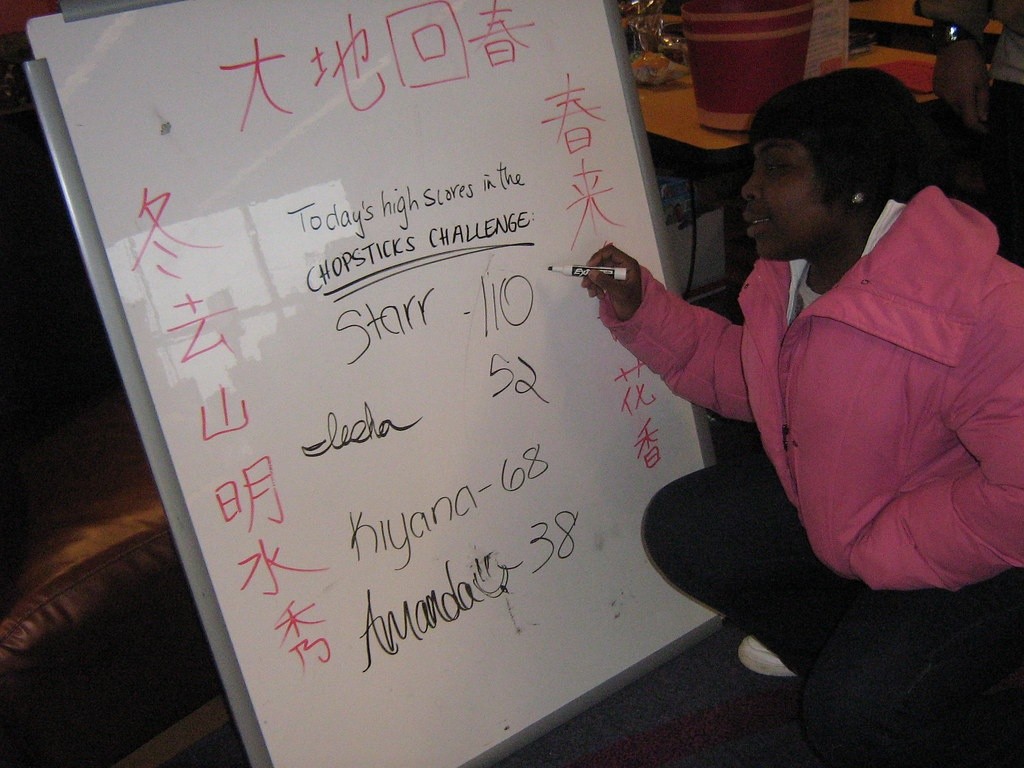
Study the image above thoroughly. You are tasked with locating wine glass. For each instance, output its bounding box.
[638,5,663,54]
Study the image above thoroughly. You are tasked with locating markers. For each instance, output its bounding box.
[548,265,627,280]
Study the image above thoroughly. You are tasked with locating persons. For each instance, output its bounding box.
[914,0,1024,268]
[575,65,1024,766]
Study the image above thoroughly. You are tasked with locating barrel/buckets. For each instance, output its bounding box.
[681,0,814,131]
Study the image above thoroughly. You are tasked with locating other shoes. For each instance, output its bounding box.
[738,635,798,676]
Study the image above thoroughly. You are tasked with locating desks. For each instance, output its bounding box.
[637,44,993,302]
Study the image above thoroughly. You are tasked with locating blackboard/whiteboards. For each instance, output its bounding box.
[21,0,759,768]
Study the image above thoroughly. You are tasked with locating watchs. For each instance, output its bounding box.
[930,21,975,45]
